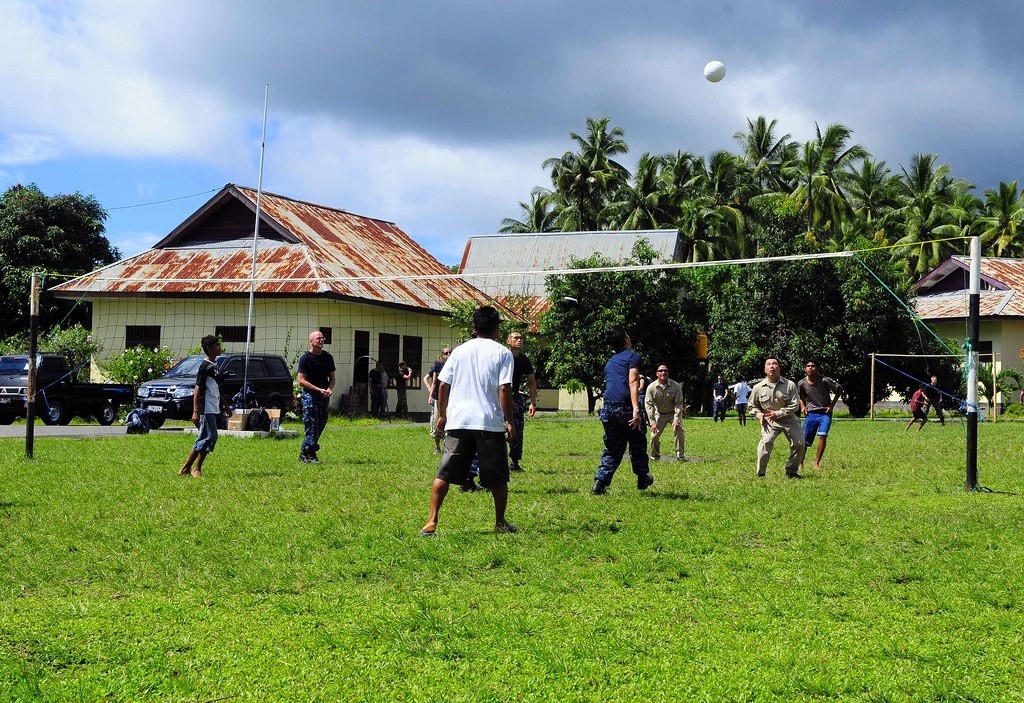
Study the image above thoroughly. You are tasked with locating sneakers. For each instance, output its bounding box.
[299,452,320,463]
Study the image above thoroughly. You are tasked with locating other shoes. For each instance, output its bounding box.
[510,463,524,472]
[593,480,607,495]
[460,479,477,491]
[638,474,653,489]
[788,473,801,479]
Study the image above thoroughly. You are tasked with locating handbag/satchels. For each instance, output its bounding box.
[249,410,270,431]
[125,408,149,435]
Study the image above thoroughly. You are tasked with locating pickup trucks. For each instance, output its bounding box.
[0,353,134,426]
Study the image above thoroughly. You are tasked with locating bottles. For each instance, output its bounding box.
[271,406,279,431]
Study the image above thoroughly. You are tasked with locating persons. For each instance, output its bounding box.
[178,334,232,477]
[418,306,518,534]
[798,361,843,471]
[920,376,945,426]
[502,331,537,471]
[297,331,336,464]
[745,358,805,481]
[589,324,652,495]
[637,373,655,427]
[905,383,929,431]
[369,347,490,493]
[1019,389,1024,405]
[645,363,685,461]
[712,375,728,422]
[733,375,752,426]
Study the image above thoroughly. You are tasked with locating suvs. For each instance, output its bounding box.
[135,353,297,431]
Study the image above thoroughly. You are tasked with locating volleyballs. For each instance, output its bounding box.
[704,60,726,83]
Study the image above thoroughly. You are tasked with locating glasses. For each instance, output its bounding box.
[444,352,451,355]
[657,370,669,372]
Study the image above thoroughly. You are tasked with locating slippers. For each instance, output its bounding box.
[419,528,432,536]
[508,525,514,532]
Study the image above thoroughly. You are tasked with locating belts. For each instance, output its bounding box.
[604,402,632,406]
